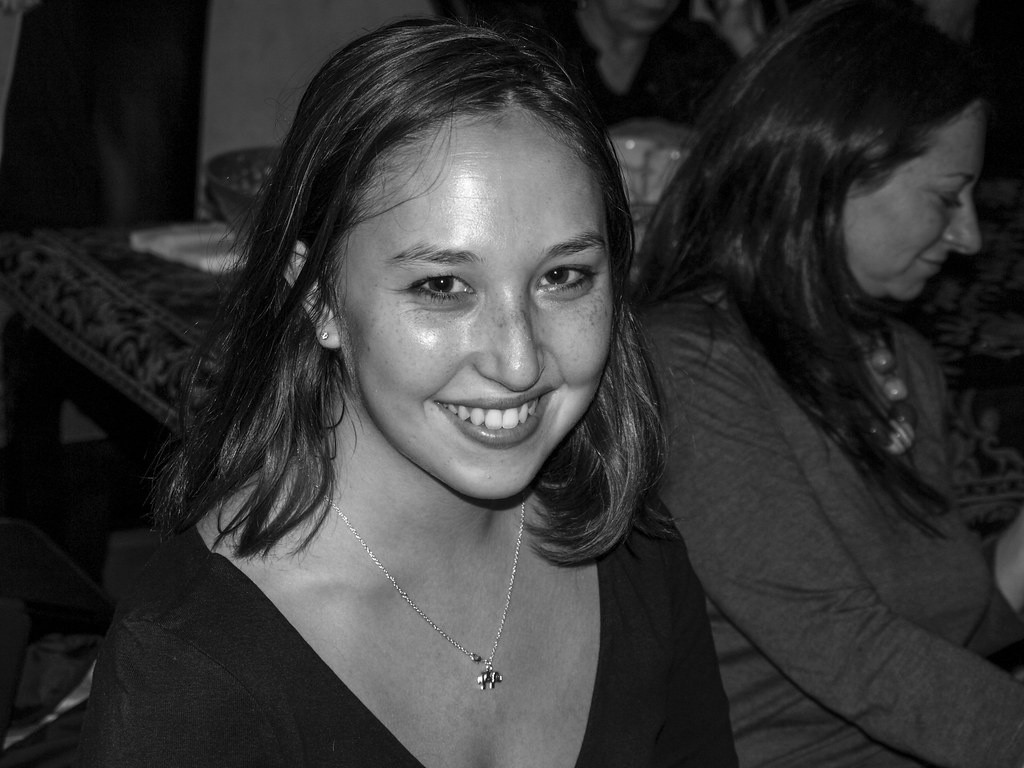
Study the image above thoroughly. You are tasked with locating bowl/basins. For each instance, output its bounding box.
[204,147,284,232]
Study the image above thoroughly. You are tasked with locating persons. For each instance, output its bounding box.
[78,0,738,768]
[639,0,1024,768]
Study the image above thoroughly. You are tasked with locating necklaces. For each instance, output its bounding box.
[311,481,524,688]
[855,317,920,453]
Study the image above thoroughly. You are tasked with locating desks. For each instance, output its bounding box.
[0,197,683,443]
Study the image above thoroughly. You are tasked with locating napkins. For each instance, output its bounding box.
[132,220,250,273]
